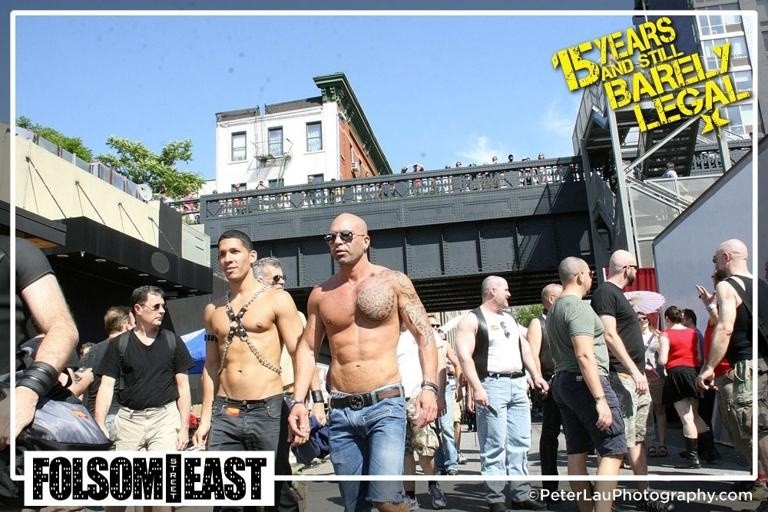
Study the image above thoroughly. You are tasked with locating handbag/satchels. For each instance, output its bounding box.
[1,366,115,455]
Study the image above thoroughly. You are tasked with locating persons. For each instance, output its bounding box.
[74,306,137,417]
[287,212,441,512]
[166,150,548,225]
[455,275,550,512]
[20,314,95,404]
[250,256,327,428]
[93,283,196,455]
[657,305,711,468]
[697,238,768,477]
[526,282,563,505]
[396,320,448,512]
[438,328,466,464]
[428,315,464,475]
[661,161,678,178]
[678,309,721,462]
[590,249,676,512]
[695,268,768,500]
[191,229,312,512]
[636,312,670,457]
[544,256,629,512]
[0,233,80,452]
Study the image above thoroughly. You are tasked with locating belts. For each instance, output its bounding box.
[480,370,527,380]
[329,384,406,409]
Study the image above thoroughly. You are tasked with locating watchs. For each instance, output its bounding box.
[289,400,304,407]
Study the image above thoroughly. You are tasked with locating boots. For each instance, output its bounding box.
[695,431,723,465]
[674,437,702,469]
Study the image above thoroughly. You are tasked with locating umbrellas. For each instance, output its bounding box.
[622,290,666,315]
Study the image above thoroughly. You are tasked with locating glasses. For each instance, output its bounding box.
[140,302,167,312]
[638,315,646,324]
[322,230,366,245]
[430,324,440,330]
[710,254,724,262]
[622,264,639,271]
[263,273,287,283]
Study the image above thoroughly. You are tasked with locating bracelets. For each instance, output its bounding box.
[420,381,440,395]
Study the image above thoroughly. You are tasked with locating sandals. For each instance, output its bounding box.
[647,445,657,457]
[658,445,669,457]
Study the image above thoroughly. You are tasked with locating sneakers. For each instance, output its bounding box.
[457,451,467,464]
[635,494,675,512]
[740,482,768,503]
[406,497,420,511]
[446,469,458,475]
[426,481,448,509]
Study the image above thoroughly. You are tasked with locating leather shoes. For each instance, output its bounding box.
[510,497,545,510]
[488,501,507,512]
